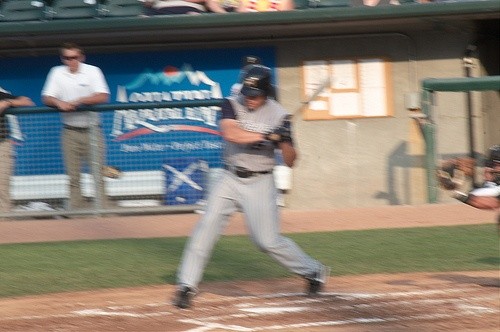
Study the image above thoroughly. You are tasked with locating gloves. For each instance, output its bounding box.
[282,114,292,128]
[264,129,292,142]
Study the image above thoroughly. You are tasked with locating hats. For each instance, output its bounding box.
[240,68,273,97]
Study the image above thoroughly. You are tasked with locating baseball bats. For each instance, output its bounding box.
[250,79,332,149]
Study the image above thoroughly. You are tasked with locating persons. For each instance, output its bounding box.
[0,87,36,220]
[138,0,433,16]
[173,64,327,309]
[41,43,110,218]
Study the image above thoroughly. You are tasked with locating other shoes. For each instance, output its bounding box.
[172,288,194,308]
[305,267,325,293]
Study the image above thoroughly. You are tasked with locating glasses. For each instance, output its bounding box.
[61,56,77,60]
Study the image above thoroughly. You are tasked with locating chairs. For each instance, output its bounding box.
[0,0,142,20]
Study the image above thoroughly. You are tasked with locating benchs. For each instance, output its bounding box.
[10,170,166,209]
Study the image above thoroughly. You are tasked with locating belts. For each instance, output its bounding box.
[63,124,99,131]
[225,164,273,178]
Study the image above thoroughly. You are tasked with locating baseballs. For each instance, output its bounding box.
[101,166,120,178]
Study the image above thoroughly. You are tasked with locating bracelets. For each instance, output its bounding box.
[264,132,273,140]
